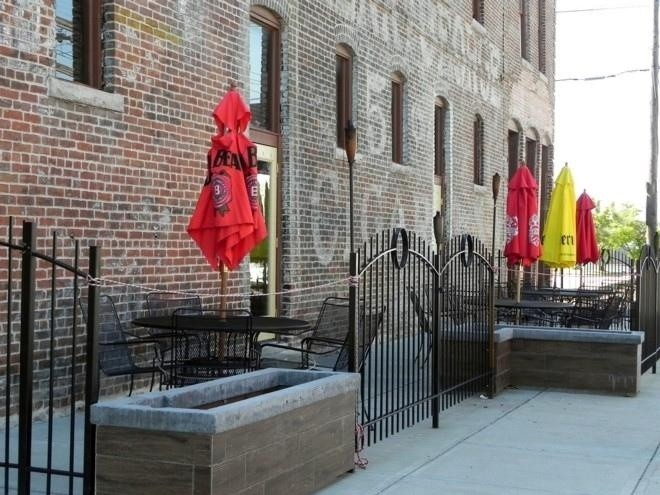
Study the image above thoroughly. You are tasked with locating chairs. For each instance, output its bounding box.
[300,305,388,431]
[253,296,365,371]
[79,294,201,398]
[165,307,261,391]
[406,278,635,370]
[145,291,211,393]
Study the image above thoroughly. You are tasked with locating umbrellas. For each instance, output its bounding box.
[503,160,542,325]
[185,83,269,381]
[576,188,601,292]
[539,158,579,306]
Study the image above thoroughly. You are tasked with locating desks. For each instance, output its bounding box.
[130,315,313,389]
